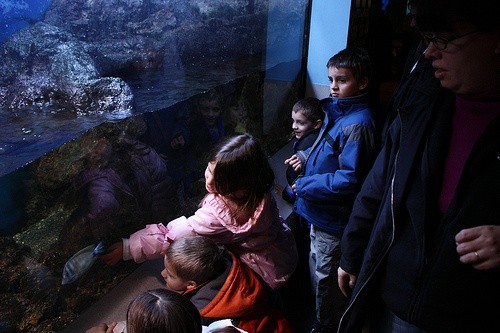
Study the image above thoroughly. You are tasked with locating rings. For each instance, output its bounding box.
[475,251,480,260]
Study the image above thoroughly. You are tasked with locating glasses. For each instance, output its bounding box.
[422,26,482,51]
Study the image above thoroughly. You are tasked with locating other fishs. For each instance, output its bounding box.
[61,241,104,285]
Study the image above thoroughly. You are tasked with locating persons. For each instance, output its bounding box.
[98,132,298,333]
[338,0,500,333]
[86,288,242,333]
[282,48,378,333]
[79,92,225,247]
[279,97,325,277]
[160,237,292,333]
